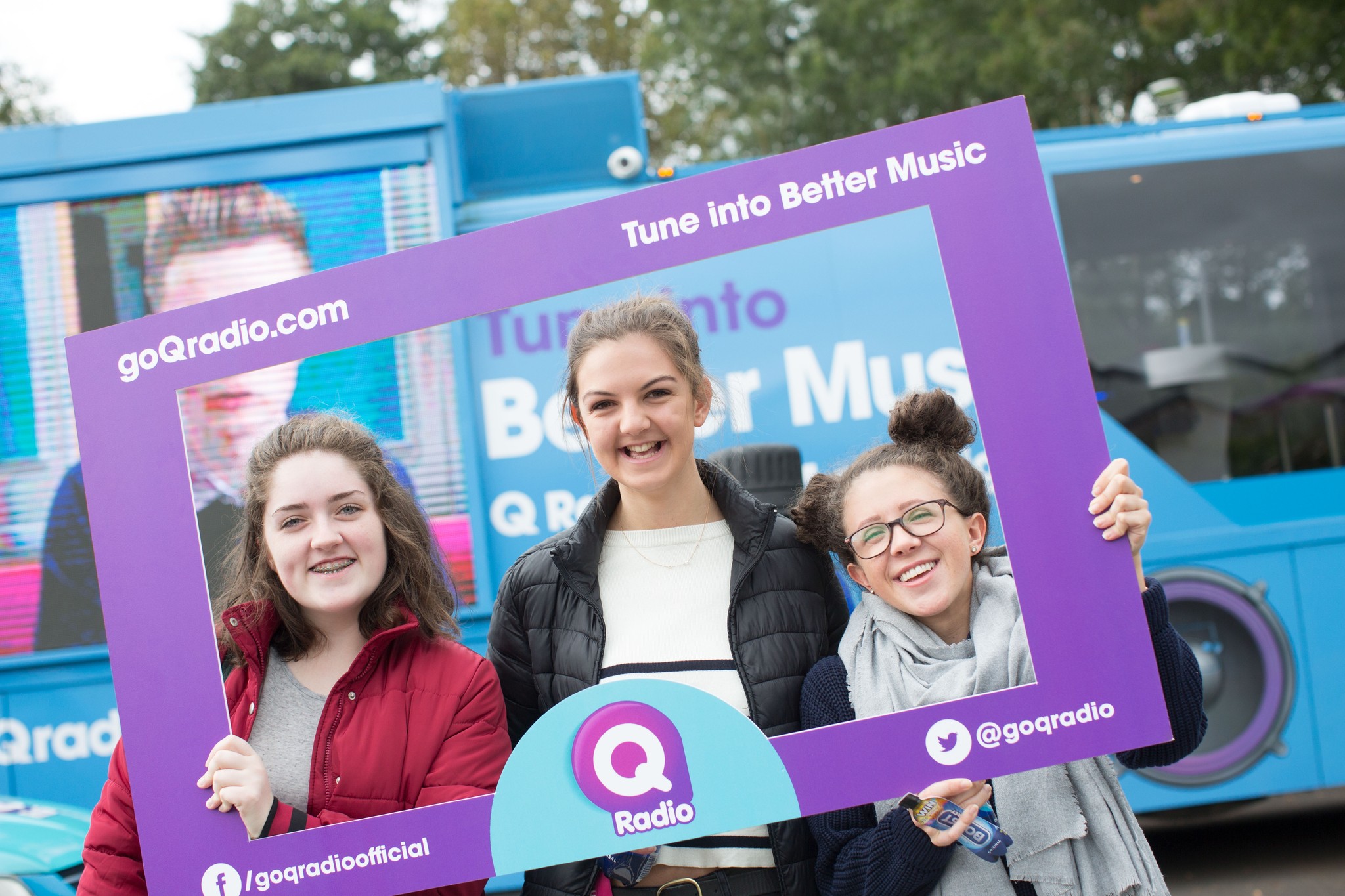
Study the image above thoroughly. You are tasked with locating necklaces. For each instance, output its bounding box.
[615,486,709,568]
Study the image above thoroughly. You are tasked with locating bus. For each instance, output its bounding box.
[451,82,1345,896]
[0,71,649,813]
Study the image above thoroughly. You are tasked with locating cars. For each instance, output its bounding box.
[0,793,93,896]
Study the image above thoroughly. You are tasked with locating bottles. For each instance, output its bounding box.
[897,793,1014,863]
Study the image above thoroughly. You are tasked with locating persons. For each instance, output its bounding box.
[478,294,862,896]
[77,410,510,896]
[27,184,314,653]
[799,388,1207,896]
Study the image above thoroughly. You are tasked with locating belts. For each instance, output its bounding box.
[607,865,781,896]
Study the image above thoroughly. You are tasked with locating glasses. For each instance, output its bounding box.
[844,498,966,559]
